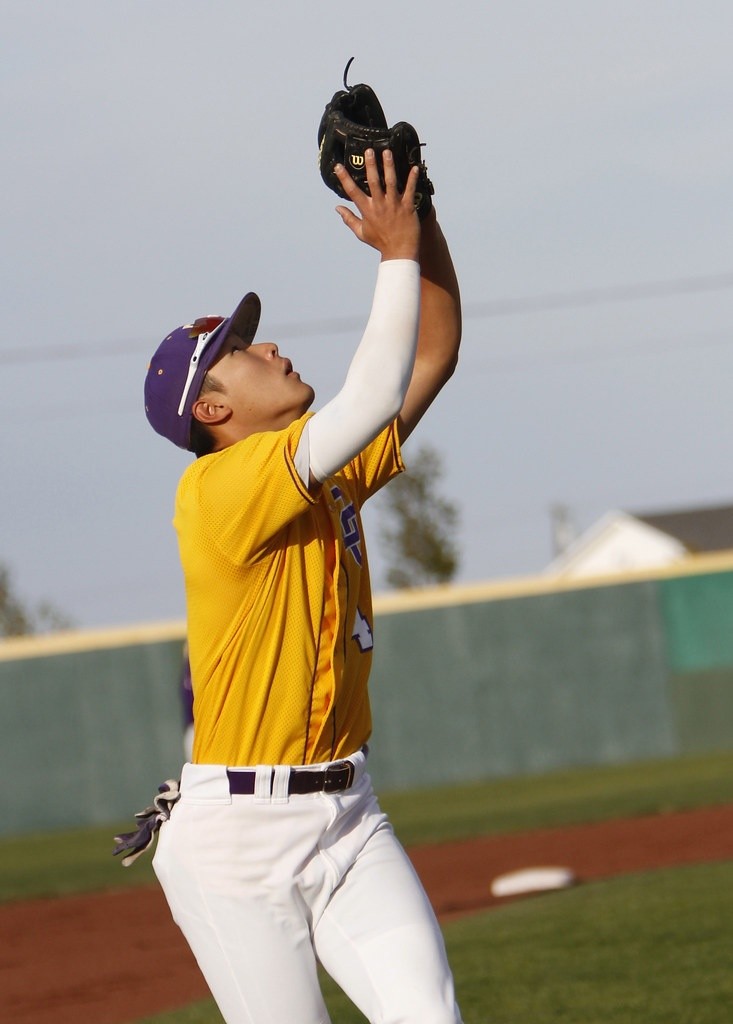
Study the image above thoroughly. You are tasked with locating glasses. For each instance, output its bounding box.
[179,314,228,415]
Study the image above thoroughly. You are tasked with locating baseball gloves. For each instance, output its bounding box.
[316,54,436,224]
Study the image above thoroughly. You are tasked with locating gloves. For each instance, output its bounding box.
[111,778,182,868]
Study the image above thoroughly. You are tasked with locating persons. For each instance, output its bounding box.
[112,148,518,1024]
[176,651,199,762]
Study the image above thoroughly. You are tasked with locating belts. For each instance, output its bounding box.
[227,742,369,795]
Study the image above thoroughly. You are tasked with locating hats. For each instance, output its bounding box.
[143,291,261,450]
